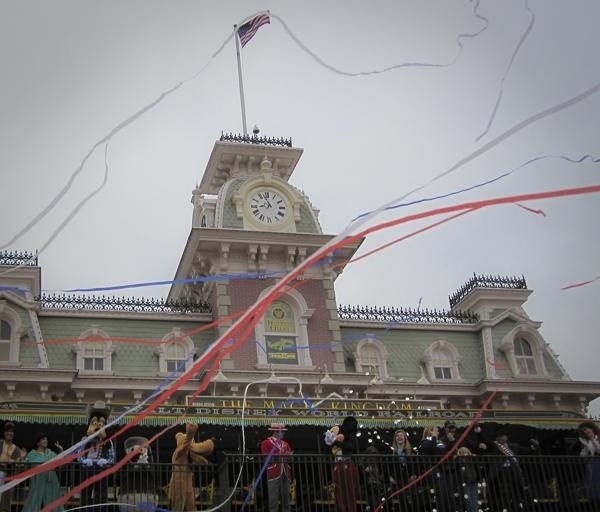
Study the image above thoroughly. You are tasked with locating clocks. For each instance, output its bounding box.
[244,186,294,230]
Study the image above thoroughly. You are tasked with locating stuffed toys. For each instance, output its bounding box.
[76,400,116,512]
[323,416,358,512]
[118,437,154,495]
[167,421,216,512]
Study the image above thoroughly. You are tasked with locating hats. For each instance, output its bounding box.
[268,423,288,430]
[4,423,17,431]
[445,420,455,428]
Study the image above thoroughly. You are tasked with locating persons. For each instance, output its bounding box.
[0,423,22,504]
[261,422,295,512]
[577,421,600,512]
[14,431,66,512]
[360,420,540,512]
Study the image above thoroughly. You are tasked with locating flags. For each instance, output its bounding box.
[238,9,270,49]
